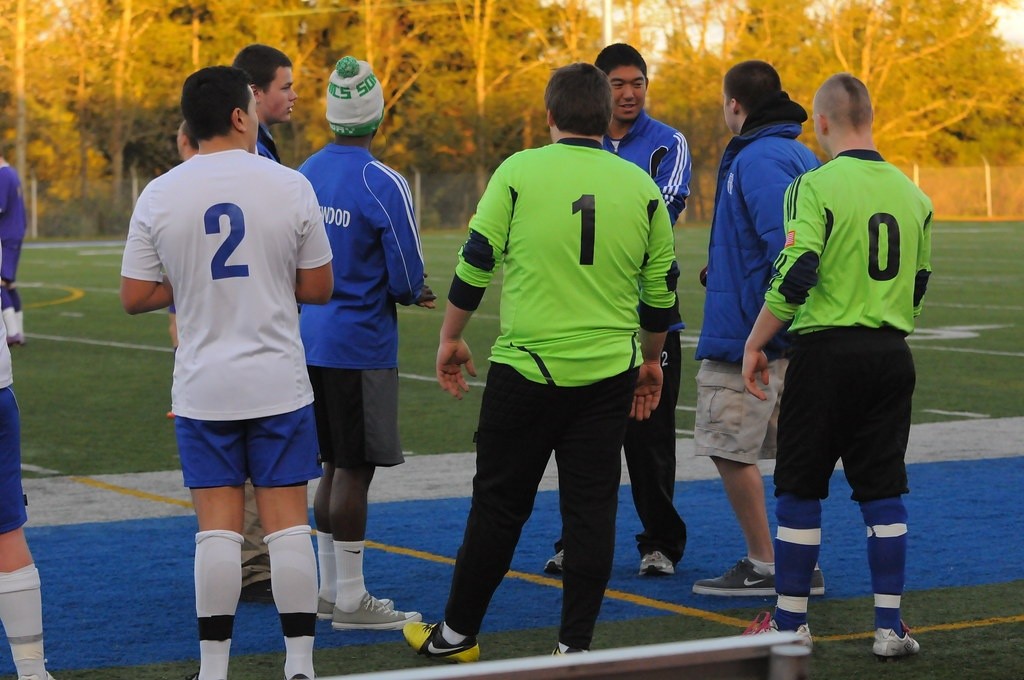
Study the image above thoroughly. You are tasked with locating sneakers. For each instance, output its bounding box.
[318,597,395,620]
[244,578,275,602]
[809,569,824,595]
[403,621,480,665]
[744,610,813,656]
[693,559,779,595]
[638,551,673,576]
[873,619,920,656]
[543,549,564,574]
[553,646,585,654]
[332,592,421,630]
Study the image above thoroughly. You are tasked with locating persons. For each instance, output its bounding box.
[691,60,824,596]
[0,238,56,680]
[742,73,934,657]
[403,63,681,666]
[233,45,298,599]
[296,56,437,632]
[120,66,334,680]
[544,43,692,577]
[0,152,27,348]
[167,120,200,419]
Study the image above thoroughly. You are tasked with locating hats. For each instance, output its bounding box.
[324,56,384,137]
